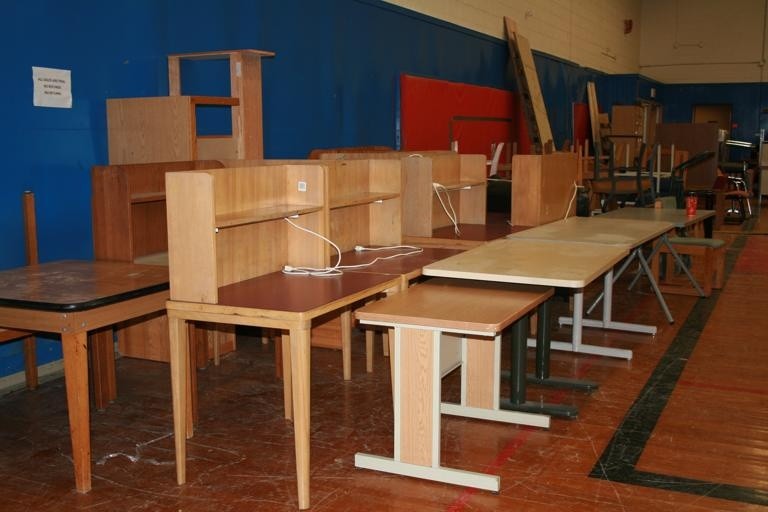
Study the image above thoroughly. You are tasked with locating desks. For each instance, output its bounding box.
[506,216,673,360]
[421,238,628,419]
[351,277,554,493]
[599,207,716,325]
[1,258,191,493]
[405,222,545,337]
[166,247,468,509]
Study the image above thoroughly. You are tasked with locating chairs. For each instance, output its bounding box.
[650,196,726,296]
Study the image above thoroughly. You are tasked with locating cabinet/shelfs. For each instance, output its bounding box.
[404,153,486,238]
[286,159,403,268]
[164,165,324,304]
[93,160,245,370]
